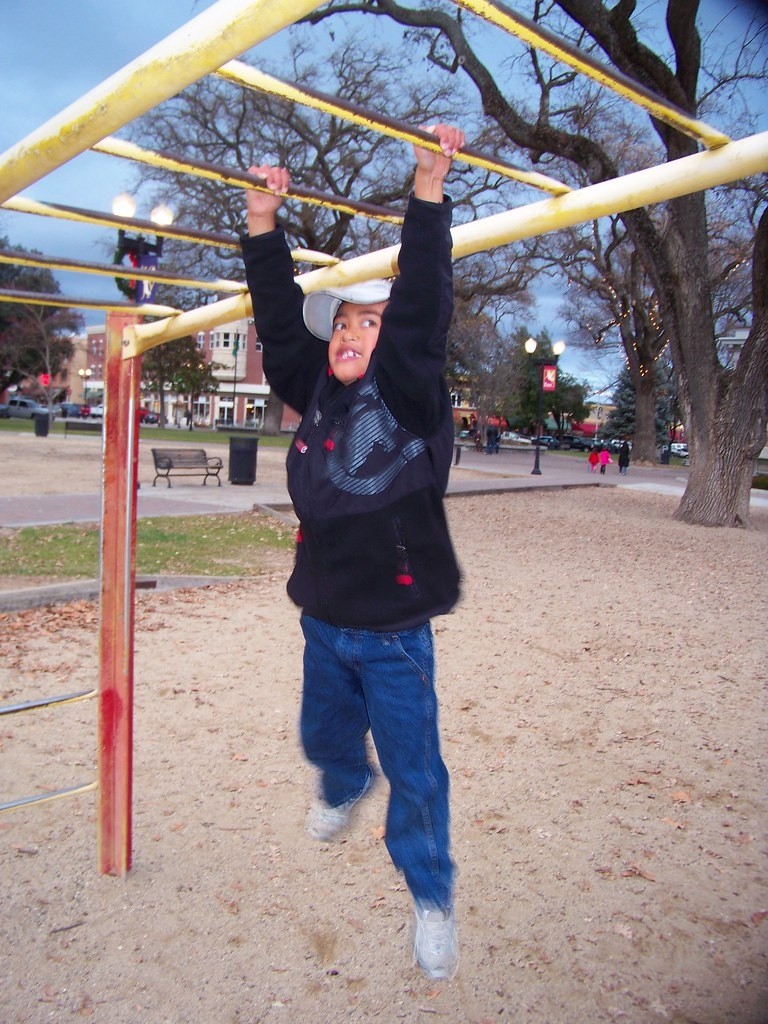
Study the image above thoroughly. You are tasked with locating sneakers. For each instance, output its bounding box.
[306,773,371,843]
[412,906,461,982]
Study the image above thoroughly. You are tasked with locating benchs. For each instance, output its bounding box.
[151,447,224,488]
[65,422,102,439]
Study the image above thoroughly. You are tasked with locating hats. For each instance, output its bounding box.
[304,280,394,342]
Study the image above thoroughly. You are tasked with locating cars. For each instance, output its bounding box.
[0,405,12,418]
[145,414,168,424]
[662,443,689,458]
[7,399,56,421]
[52,402,103,420]
[460,430,632,453]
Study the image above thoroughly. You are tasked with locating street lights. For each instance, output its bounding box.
[524,337,566,475]
[109,193,174,302]
[79,369,92,405]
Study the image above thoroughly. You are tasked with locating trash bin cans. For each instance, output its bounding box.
[35,413,48,437]
[229,436,260,486]
[661,454,669,465]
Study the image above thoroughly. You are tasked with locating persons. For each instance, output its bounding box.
[186,410,191,425]
[619,442,629,476]
[241,120,462,978]
[589,447,599,473]
[462,412,500,455]
[599,446,610,474]
